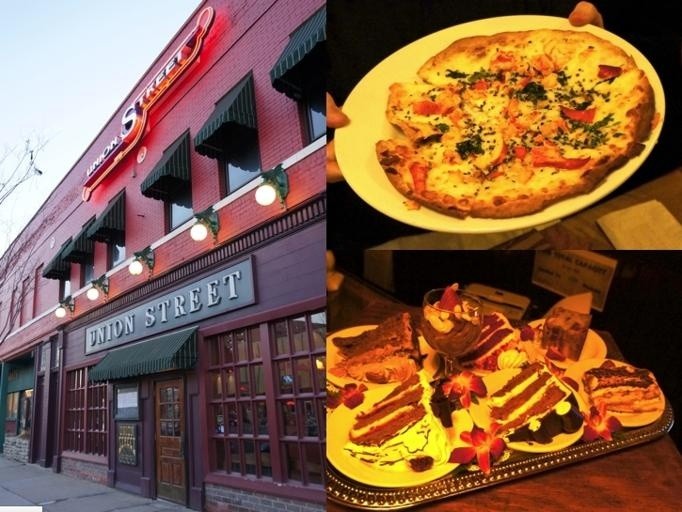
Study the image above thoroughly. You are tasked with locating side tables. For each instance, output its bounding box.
[324,268,681,512]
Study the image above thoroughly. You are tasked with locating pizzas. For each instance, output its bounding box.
[375,27,655,219]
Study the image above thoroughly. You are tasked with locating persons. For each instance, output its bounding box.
[567,1,606,29]
[327,88,352,187]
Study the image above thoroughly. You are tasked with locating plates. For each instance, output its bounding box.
[331,13,670,234]
[512,318,609,368]
[327,384,474,488]
[468,369,586,453]
[326,326,424,385]
[572,360,666,429]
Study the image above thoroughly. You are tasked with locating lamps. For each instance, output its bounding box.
[129,246,156,277]
[56,272,112,318]
[254,163,290,207]
[190,207,219,244]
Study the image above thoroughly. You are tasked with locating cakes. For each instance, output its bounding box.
[334,301,663,474]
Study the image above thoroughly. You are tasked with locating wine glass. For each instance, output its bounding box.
[421,286,484,385]
